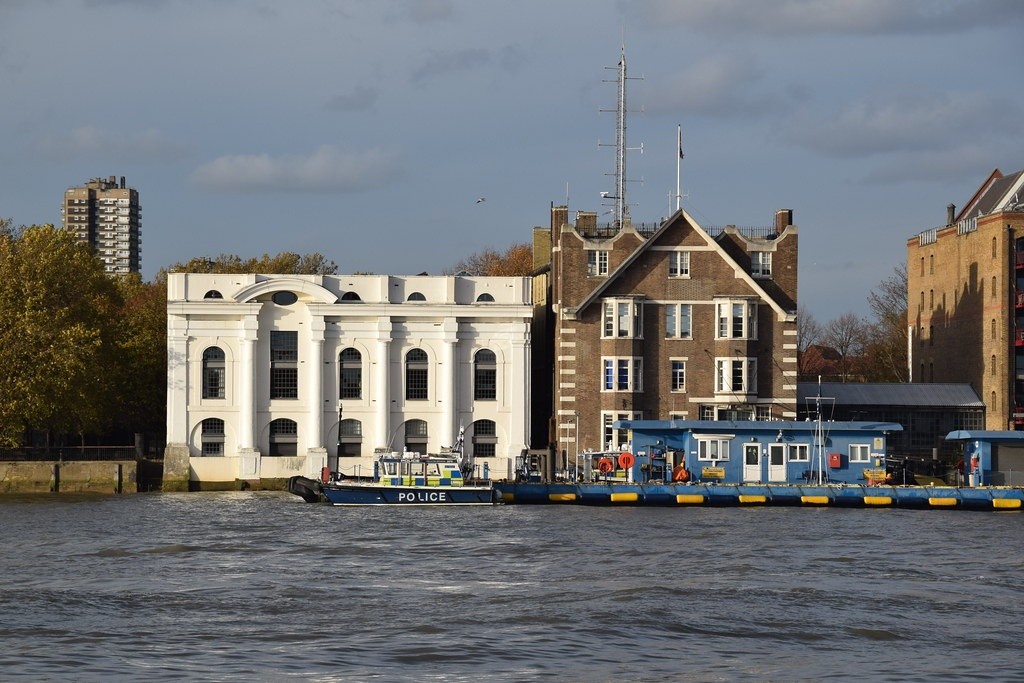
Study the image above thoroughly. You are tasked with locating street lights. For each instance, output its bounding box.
[574,412,580,482]
[336,407,342,480]
[566,418,572,471]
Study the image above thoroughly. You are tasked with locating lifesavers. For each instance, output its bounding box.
[597,458,613,473]
[618,452,634,468]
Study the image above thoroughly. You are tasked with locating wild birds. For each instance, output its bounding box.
[476,198,486,204]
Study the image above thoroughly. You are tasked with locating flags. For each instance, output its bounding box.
[680,130,684,159]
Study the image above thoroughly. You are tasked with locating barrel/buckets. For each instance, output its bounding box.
[969,473,979,486]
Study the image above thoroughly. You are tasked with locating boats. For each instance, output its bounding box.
[320,452,494,506]
